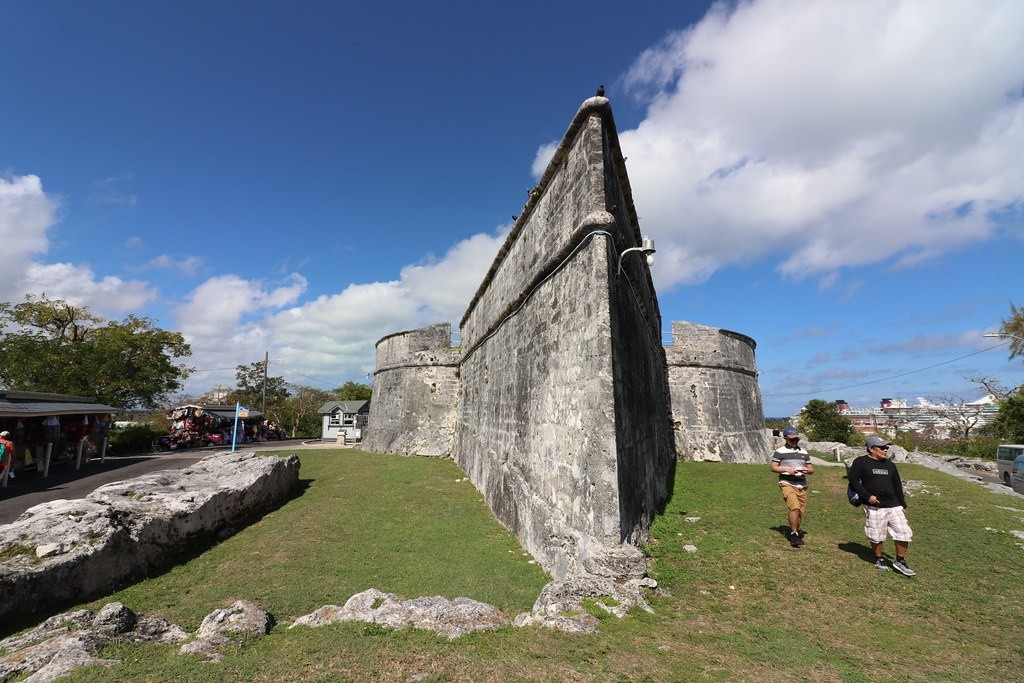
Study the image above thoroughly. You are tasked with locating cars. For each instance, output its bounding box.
[1009,455,1024,497]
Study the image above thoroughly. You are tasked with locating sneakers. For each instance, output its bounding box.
[790,533,799,546]
[798,531,804,542]
[892,559,917,576]
[875,558,889,570]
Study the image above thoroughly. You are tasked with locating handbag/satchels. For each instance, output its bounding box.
[847,476,864,508]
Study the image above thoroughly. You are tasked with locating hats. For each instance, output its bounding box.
[867,435,893,446]
[0,431,10,436]
[782,427,800,439]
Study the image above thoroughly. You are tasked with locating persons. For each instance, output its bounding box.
[771,426,815,544]
[0,430,15,489]
[847,437,916,576]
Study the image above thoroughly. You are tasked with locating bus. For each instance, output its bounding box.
[996,444,1024,487]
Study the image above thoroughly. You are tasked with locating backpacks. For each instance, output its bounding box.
[0,440,9,463]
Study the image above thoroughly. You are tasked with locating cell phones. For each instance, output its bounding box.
[796,469,808,472]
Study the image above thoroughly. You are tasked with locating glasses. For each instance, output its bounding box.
[874,446,889,450]
[787,438,800,442]
